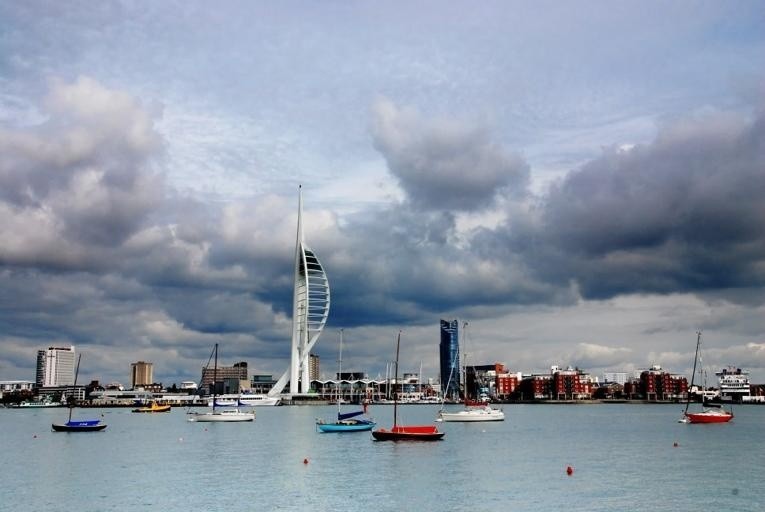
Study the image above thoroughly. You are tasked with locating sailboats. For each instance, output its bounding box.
[370,329,448,445]
[431,321,510,425]
[50,353,112,436]
[680,330,736,424]
[186,342,260,424]
[328,357,452,406]
[311,325,378,437]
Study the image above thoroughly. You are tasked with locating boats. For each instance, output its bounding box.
[6,386,287,416]
